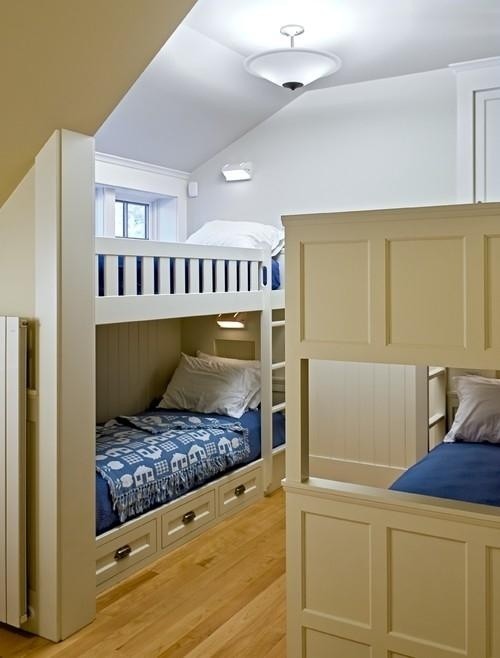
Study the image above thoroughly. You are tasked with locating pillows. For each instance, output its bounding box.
[182,220,284,259]
[194,350,263,410]
[442,375,500,445]
[156,352,262,419]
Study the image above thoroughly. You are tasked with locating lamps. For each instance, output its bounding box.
[243,26,342,92]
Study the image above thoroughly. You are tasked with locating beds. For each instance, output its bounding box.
[280,202,500,657]
[1,128,286,644]
